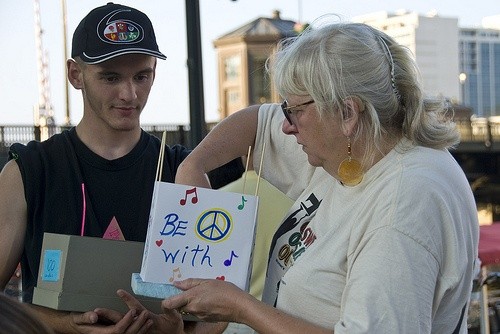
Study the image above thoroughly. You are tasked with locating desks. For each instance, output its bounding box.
[477,222,500,334]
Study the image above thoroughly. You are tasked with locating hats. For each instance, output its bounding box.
[72,2,167,65]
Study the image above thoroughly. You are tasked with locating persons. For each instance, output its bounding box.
[162,23,480,334]
[0,2,230,334]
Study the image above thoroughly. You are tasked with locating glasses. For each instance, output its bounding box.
[280,100,316,125]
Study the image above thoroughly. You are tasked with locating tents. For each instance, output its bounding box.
[477,222,500,334]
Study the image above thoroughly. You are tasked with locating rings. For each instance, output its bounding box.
[179,308,190,315]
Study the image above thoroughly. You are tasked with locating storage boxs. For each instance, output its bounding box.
[32,232,168,313]
